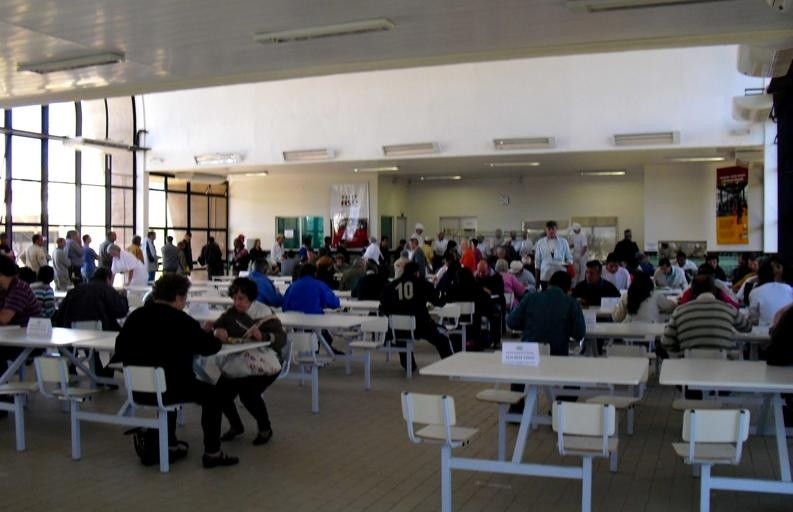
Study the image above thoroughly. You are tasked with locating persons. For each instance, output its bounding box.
[2,221,792,422]
[213,278,288,445]
[114,274,239,468]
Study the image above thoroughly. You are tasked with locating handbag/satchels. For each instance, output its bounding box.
[219,346,281,377]
[122,424,160,468]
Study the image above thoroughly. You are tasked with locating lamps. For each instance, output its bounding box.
[279,147,337,163]
[13,50,126,76]
[613,128,673,148]
[488,134,558,152]
[381,141,442,159]
[250,16,394,48]
[191,152,243,168]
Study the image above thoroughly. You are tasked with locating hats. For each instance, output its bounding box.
[508,260,523,275]
[572,222,581,231]
[414,223,424,230]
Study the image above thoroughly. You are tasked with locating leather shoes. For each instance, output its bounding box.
[201,453,239,471]
[249,427,273,448]
[220,423,246,442]
[167,440,190,460]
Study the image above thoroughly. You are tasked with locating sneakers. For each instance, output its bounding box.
[332,347,344,355]
